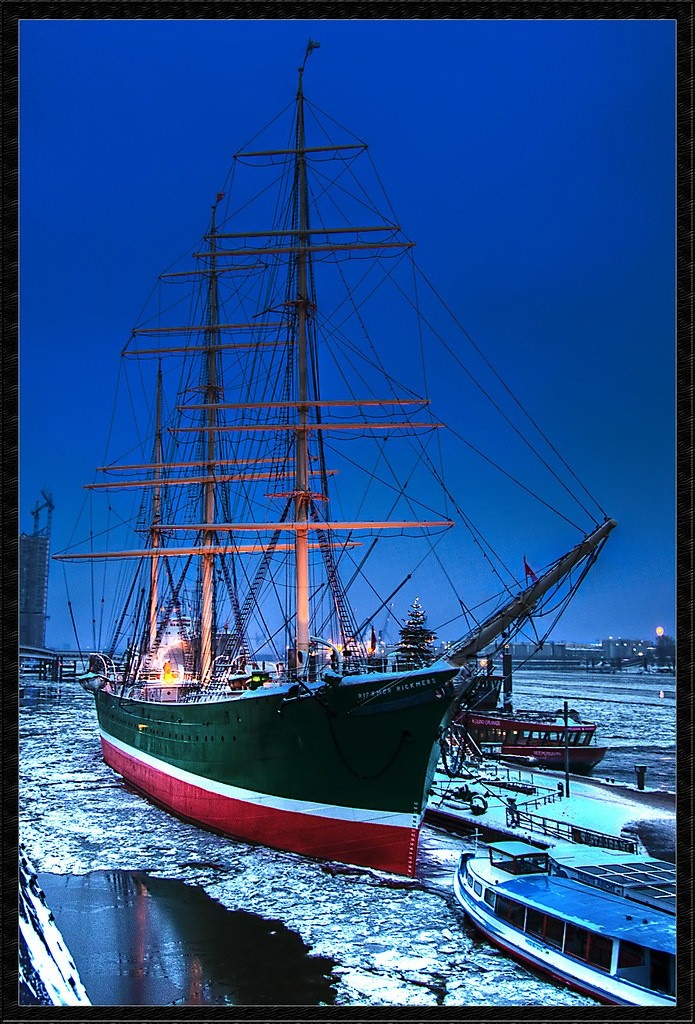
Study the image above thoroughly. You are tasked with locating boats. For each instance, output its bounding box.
[452,837,677,1008]
[414,598,610,776]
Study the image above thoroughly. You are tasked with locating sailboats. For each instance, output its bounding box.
[48,35,475,890]
